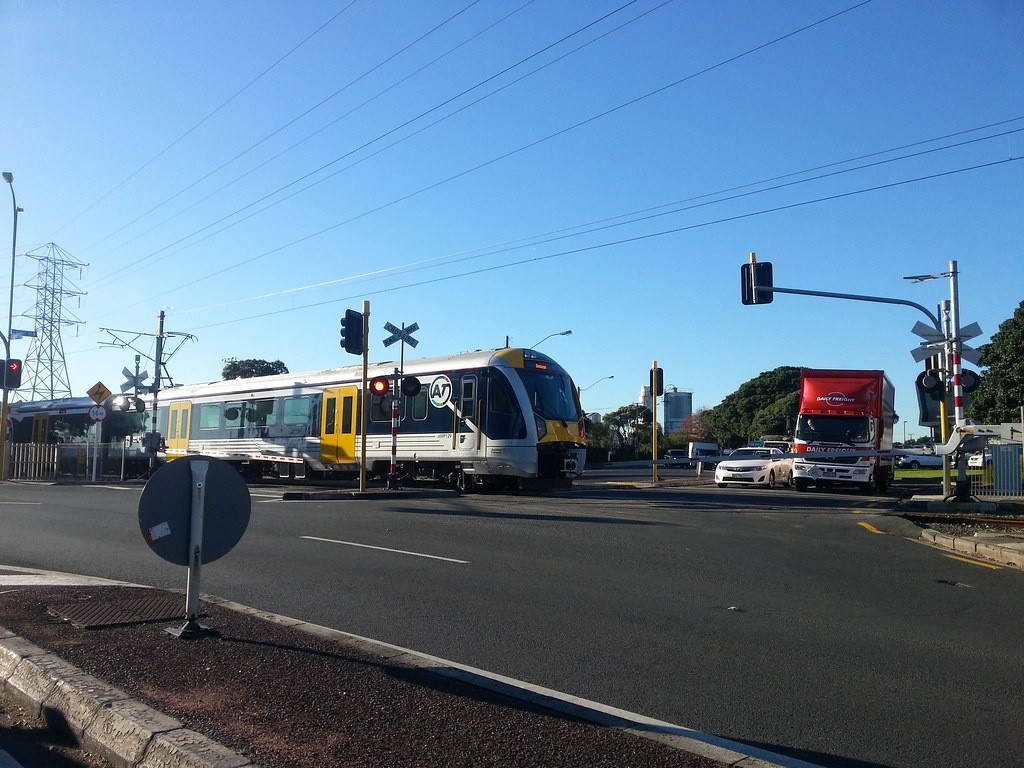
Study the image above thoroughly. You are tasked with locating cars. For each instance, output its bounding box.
[714,447,793,489]
[721,439,793,454]
[896,442,994,471]
[664,448,689,468]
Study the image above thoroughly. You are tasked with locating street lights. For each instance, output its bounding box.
[577,374,615,398]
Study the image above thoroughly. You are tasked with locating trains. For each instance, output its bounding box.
[6,346,588,497]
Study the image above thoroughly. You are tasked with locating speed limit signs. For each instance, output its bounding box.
[89,406,107,422]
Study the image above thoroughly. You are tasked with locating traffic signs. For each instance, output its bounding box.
[12,329,37,337]
[383,322,420,348]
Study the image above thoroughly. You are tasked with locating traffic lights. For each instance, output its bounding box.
[340,309,364,356]
[369,376,421,397]
[6,359,21,389]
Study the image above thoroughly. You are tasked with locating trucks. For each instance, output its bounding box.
[689,442,720,470]
[792,366,897,496]
